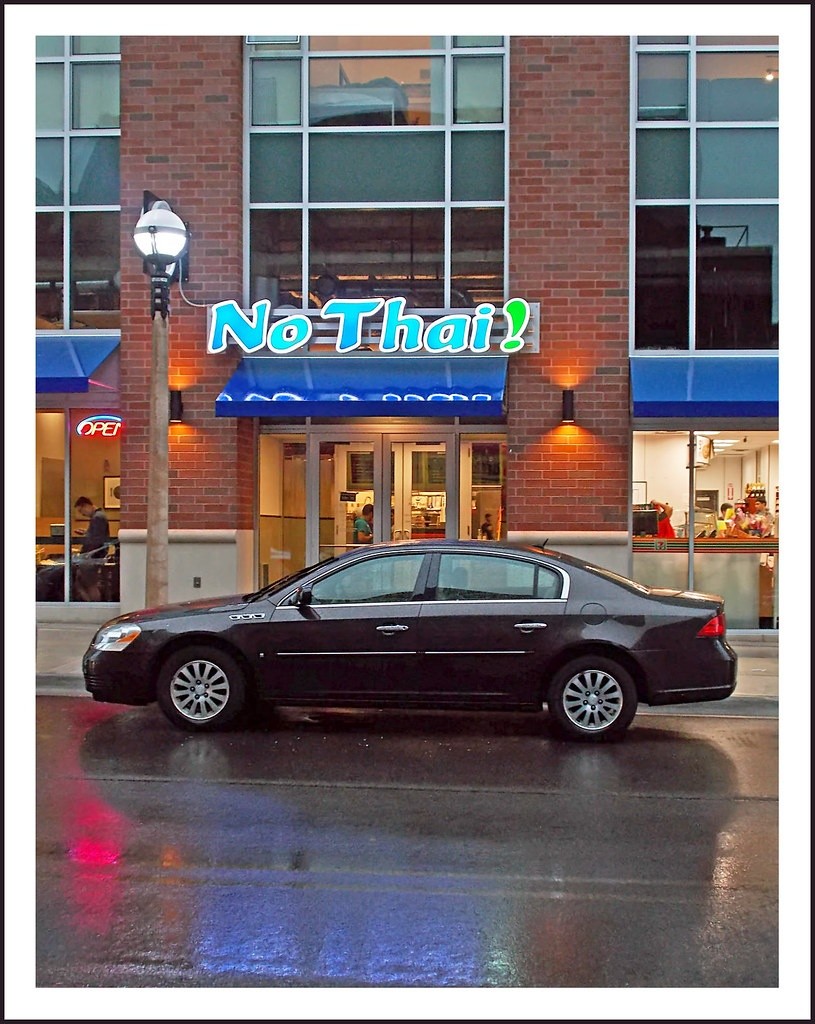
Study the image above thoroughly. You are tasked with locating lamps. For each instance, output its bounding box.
[562,390,574,422]
[169,390,184,423]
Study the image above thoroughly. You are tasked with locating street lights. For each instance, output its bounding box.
[134,198,190,612]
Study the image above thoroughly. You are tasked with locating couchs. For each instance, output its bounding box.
[639,74,777,119]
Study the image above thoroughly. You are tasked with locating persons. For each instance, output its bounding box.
[644,496,779,540]
[73,496,110,602]
[352,504,395,549]
[482,514,494,540]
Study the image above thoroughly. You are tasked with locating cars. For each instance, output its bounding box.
[82,539,738,744]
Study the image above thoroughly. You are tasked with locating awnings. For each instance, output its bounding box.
[36,336,123,393]
[628,356,779,417]
[214,359,507,417]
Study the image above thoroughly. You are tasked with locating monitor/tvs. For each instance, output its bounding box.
[632,509,658,538]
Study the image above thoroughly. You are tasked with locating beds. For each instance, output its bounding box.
[310,87,410,126]
